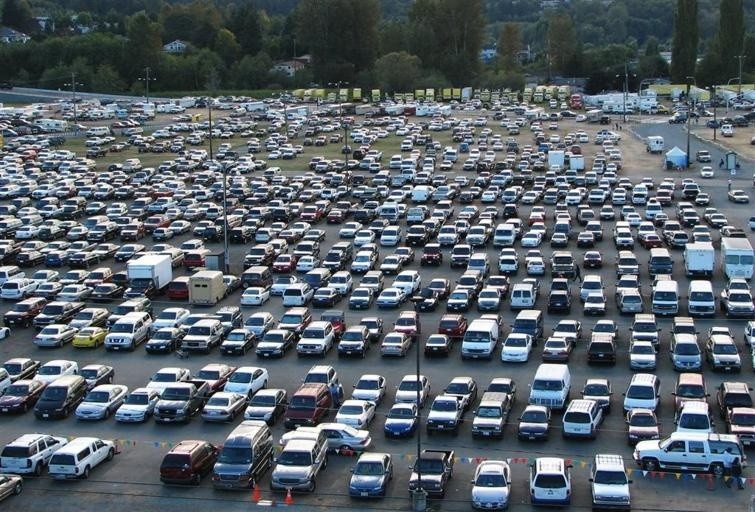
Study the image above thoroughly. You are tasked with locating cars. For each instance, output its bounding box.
[0,82,14,90]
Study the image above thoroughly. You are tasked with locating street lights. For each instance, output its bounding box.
[686,76,698,111]
[734,54,748,98]
[136,66,157,104]
[62,71,85,125]
[727,76,740,118]
[616,73,640,102]
